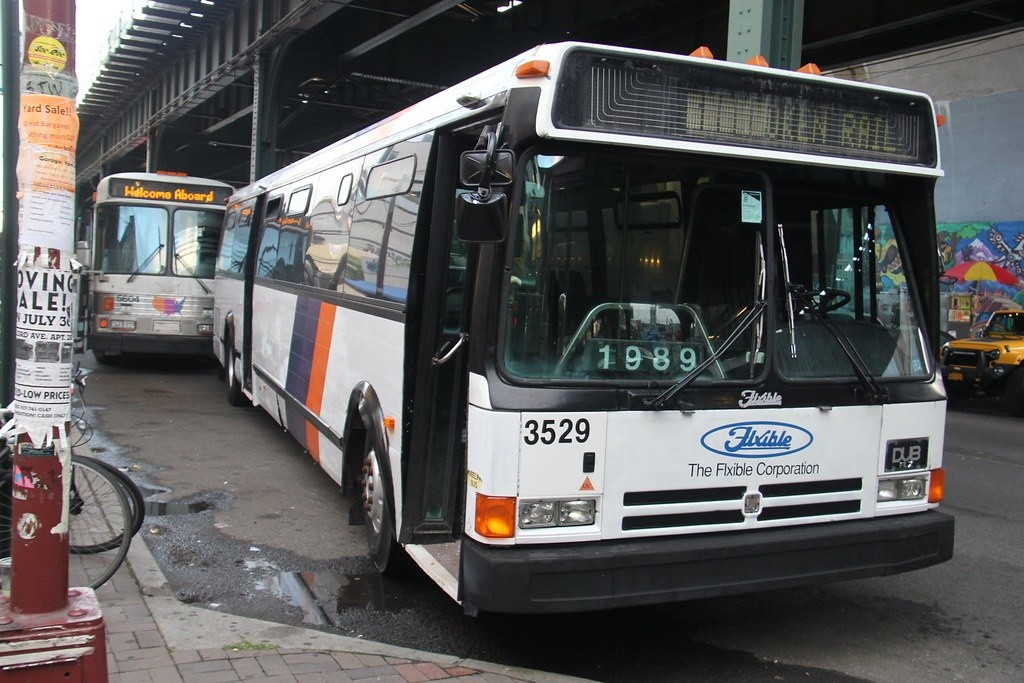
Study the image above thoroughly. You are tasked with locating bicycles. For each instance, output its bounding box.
[0,362,146,590]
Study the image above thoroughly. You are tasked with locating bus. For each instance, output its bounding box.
[78,171,236,363]
[214,41,956,620]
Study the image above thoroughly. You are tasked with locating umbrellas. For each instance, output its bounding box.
[941,260,1021,325]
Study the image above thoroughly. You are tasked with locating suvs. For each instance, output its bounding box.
[939,309,1023,415]
